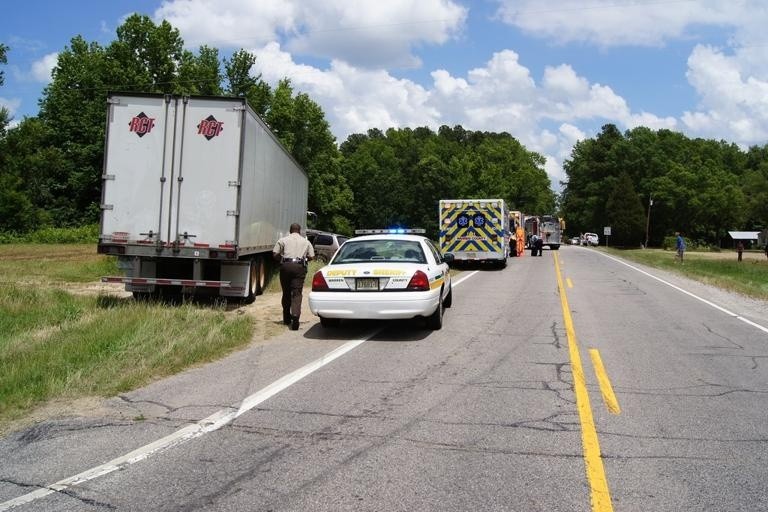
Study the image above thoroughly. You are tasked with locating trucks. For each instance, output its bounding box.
[438,198,562,268]
[97,91,309,304]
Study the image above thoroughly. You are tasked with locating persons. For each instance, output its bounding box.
[736,241,744,262]
[529,235,543,256]
[508,231,517,256]
[675,232,686,265]
[587,234,594,247]
[272,223,315,330]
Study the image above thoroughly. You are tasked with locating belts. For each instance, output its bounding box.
[283,258,307,265]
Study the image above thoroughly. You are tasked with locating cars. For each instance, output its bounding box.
[568,233,599,247]
[308,229,452,329]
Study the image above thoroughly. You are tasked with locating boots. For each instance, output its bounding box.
[284,314,299,330]
